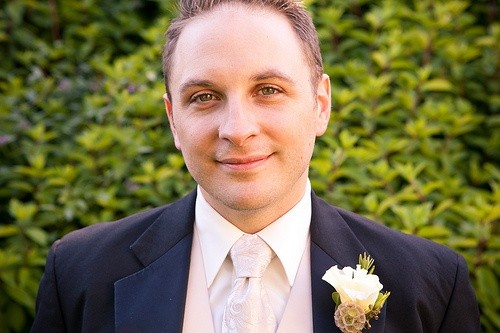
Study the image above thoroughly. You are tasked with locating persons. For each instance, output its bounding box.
[31,0,483,333]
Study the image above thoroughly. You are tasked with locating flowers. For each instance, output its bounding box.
[321,251,392,333]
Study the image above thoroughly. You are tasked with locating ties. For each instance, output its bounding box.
[221,234,276,333]
